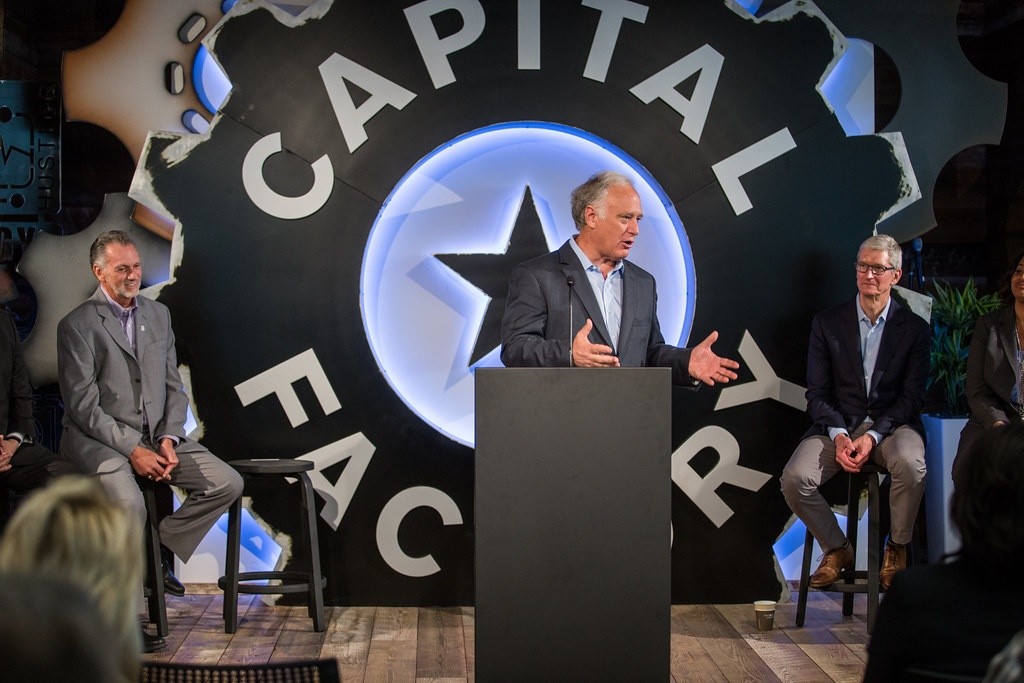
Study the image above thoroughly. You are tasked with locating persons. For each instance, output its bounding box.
[53,230,245,652]
[781,234,930,587]
[864,255,1024,683]
[0,305,149,683]
[500,169,742,388]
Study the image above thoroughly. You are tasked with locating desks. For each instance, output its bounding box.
[921,413,970,564]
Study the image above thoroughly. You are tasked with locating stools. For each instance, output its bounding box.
[217,458,328,633]
[790,464,921,632]
[133,474,171,637]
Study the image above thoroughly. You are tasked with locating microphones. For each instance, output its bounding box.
[913,237,925,291]
[567,275,575,368]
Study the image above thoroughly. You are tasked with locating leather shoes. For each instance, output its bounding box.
[144,547,185,598]
[141,629,167,651]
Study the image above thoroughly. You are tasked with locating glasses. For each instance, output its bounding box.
[853,261,895,275]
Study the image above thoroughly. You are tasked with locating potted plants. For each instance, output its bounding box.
[927,274,1004,419]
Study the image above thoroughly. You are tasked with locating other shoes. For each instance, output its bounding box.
[809,540,854,587]
[881,533,906,592]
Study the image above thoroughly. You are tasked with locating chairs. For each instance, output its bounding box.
[137,657,342,683]
